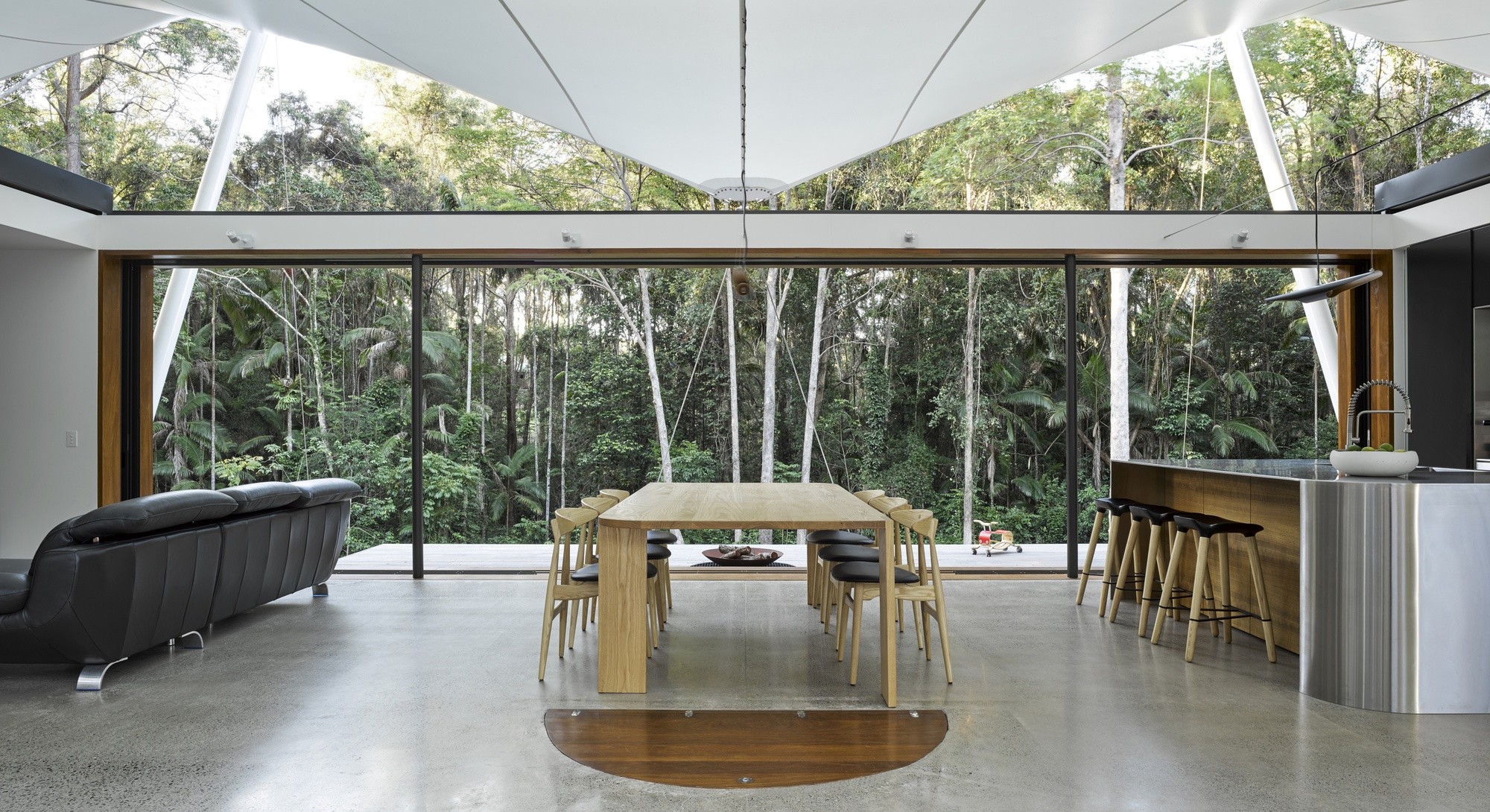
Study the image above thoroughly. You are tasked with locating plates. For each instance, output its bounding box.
[702,548,784,566]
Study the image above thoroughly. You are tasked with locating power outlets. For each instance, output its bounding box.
[66,431,77,446]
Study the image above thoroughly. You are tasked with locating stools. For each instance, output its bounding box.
[1076,496,1277,665]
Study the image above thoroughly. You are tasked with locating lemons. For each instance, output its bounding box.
[1346,444,1406,452]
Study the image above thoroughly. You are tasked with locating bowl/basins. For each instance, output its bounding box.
[1329,450,1419,476]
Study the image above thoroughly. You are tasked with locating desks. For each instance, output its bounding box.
[599,482,896,707]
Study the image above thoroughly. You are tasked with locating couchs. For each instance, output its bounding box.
[0,478,362,691]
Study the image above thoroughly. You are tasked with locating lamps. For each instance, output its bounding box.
[1265,89,1490,303]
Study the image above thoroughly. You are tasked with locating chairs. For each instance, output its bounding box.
[536,489,675,681]
[807,490,952,687]
